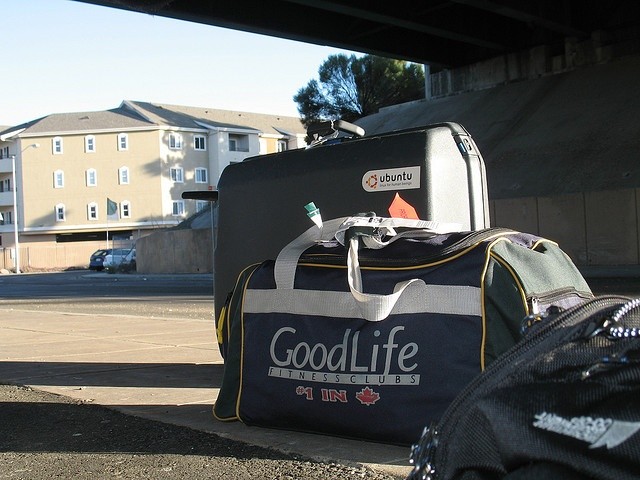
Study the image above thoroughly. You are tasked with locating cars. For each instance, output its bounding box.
[103,249,136,271]
[89,248,116,270]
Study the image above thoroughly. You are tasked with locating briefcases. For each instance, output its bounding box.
[214,120,491,325]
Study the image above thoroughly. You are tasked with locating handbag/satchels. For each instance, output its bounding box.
[212,216,593,449]
[408,296,640,479]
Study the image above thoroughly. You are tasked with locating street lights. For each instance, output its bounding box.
[0,143,40,274]
